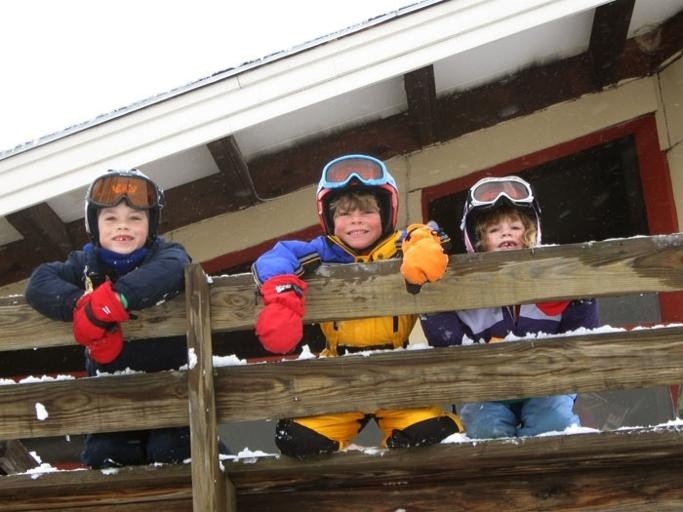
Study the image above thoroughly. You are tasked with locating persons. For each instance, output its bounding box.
[415,173,601,443]
[23,166,233,471]
[247,154,465,459]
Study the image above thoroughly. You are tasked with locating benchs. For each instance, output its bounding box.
[0,235,683,511]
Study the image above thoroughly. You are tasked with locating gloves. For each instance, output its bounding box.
[254,274,307,355]
[73,280,129,364]
[399,224,447,294]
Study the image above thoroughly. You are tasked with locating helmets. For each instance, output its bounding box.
[460,175,543,252]
[316,154,399,234]
[82,167,165,247]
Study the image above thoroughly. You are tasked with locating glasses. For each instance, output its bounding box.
[87,172,158,208]
[317,154,400,193]
[466,175,536,205]
